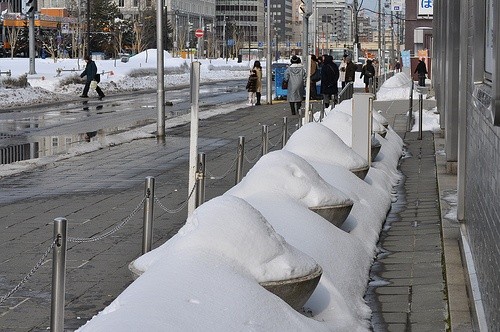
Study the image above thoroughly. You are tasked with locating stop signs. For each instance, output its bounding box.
[195,29,204,37]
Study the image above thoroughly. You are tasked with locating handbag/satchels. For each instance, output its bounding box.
[94,74,100,82]
[310,69,321,83]
[282,79,288,89]
[89,81,97,90]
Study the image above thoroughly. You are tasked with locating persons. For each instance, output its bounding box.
[283,56,307,115]
[78,56,106,100]
[414,60,429,86]
[310,53,402,109]
[252,60,263,105]
[245,70,258,106]
[82,101,103,143]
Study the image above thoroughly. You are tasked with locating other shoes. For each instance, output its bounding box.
[79,94,88,97]
[98,94,105,100]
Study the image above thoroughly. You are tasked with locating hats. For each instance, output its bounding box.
[291,55,300,64]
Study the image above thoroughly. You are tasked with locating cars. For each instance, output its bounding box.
[272,62,288,79]
